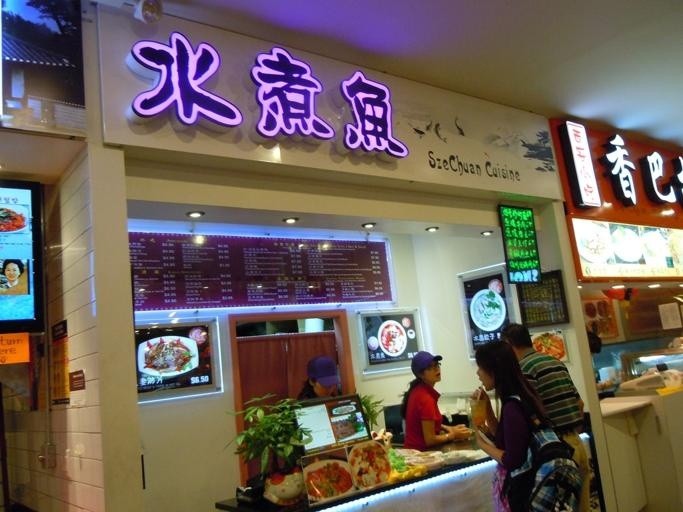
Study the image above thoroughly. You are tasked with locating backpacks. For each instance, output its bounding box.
[498,394,582,512]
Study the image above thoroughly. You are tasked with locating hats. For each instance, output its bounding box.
[411,351,441,373]
[307,356,338,388]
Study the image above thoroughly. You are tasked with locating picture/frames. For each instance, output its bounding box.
[514,269,570,328]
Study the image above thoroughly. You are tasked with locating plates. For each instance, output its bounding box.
[136,335,200,377]
[347,439,392,491]
[0,203,29,234]
[301,458,355,503]
[642,230,670,265]
[376,319,407,357]
[575,222,612,264]
[611,227,642,265]
[468,289,506,332]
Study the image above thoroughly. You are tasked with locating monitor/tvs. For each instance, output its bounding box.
[292,393,373,457]
[0,179,49,338]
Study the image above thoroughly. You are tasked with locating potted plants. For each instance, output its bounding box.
[219,390,313,500]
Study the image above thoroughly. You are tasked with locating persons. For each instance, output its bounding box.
[1,259,27,292]
[468,340,559,512]
[296,355,339,403]
[399,350,473,453]
[500,322,595,511]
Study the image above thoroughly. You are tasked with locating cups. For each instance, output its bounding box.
[468,399,488,427]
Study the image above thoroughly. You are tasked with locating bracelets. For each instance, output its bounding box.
[446,434,450,442]
